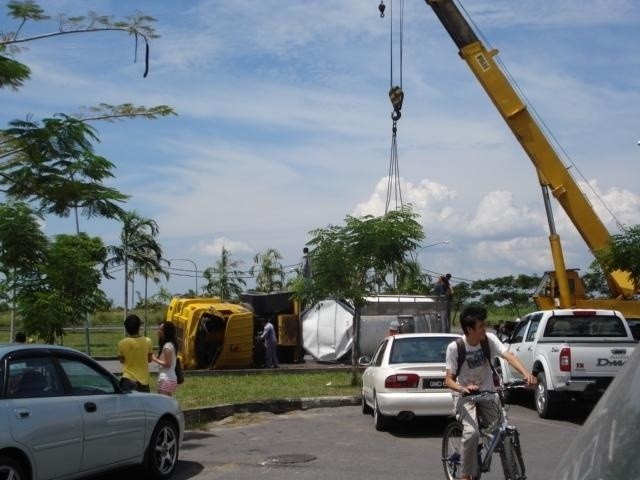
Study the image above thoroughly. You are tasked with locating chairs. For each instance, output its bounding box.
[551,320,574,334]
[589,321,612,335]
[420,349,442,362]
[14,371,47,396]
[393,351,418,362]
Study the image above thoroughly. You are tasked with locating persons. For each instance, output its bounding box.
[445,306,538,480]
[15,332,26,344]
[434,273,452,296]
[389,320,400,336]
[301,247,311,277]
[152,322,178,397]
[255,318,279,368]
[117,315,153,393]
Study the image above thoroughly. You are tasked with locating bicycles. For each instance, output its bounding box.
[441,380,526,480]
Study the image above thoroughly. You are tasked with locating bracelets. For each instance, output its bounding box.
[460,387,464,393]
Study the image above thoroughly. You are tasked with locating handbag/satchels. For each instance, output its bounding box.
[175,357,184,384]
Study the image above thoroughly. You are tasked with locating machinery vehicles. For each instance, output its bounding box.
[377,0,639,321]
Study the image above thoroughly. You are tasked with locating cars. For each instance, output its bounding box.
[358,332,462,434]
[0,344,185,480]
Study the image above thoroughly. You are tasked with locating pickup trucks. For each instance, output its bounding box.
[494,309,639,418]
